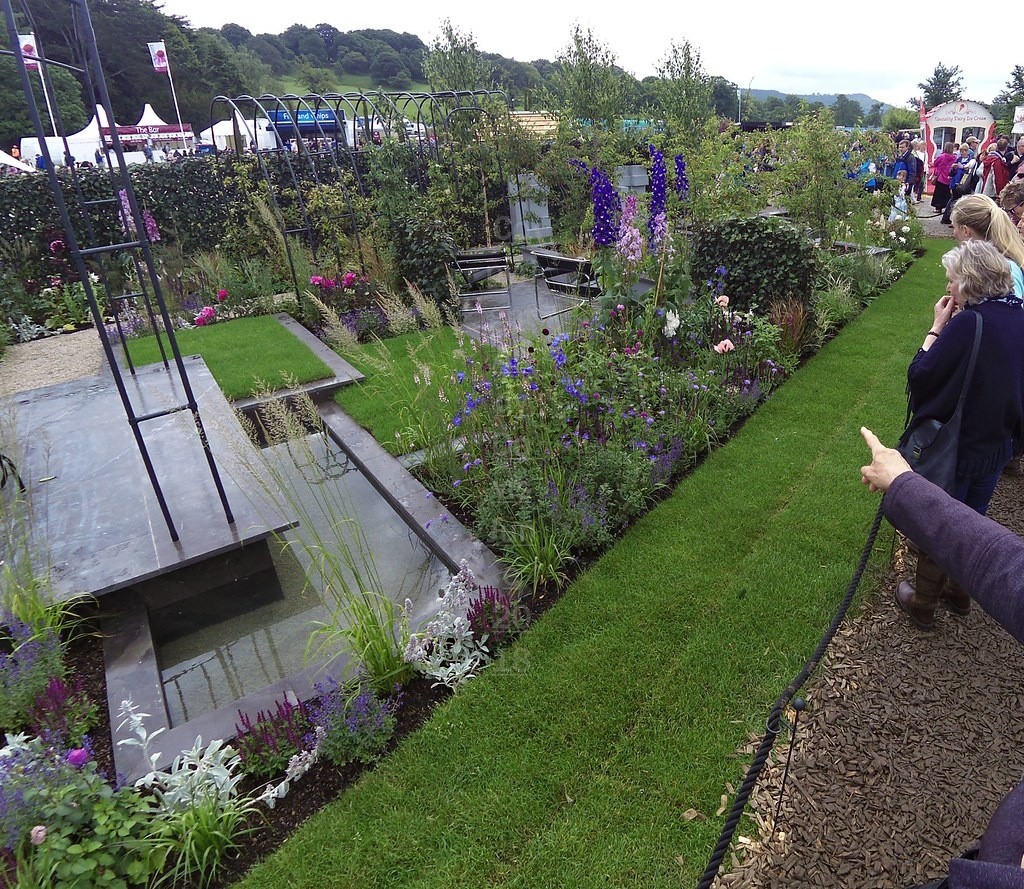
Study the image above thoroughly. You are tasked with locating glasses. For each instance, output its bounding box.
[1004,200,1024,214]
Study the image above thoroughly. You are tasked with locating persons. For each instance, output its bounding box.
[948,193,1024,476]
[11,144,20,160]
[143,141,200,165]
[896,237,1024,632]
[860,422,1024,889]
[36,154,47,169]
[63,151,75,166]
[839,129,1024,234]
[250,140,256,154]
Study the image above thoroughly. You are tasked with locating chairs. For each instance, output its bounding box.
[442,238,512,312]
[532,252,605,320]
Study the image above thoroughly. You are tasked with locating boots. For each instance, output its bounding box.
[942,581,971,615]
[896,548,948,629]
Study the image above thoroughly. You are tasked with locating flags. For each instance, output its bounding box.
[16,34,39,70]
[149,41,169,72]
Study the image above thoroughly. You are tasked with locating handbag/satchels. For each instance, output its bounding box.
[955,162,977,195]
[895,417,960,499]
[928,174,938,186]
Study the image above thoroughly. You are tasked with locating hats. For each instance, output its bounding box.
[959,142,969,151]
[966,136,981,143]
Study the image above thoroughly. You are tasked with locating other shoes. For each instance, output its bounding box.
[917,199,924,203]
[940,217,952,225]
[906,538,920,554]
[911,201,920,205]
[934,207,942,212]
[1003,455,1023,476]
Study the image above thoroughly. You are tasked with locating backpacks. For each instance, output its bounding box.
[906,154,924,183]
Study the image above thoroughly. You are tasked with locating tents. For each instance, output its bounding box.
[21,102,197,169]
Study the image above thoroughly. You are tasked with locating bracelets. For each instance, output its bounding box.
[926,331,941,338]
